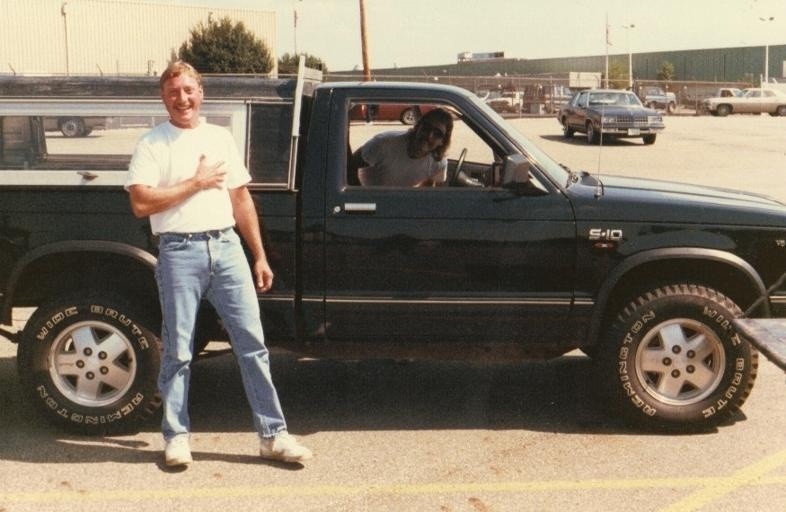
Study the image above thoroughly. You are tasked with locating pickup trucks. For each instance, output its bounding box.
[1,54,786,438]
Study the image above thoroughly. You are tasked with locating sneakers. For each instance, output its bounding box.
[259,429,313,464]
[165,438,192,467]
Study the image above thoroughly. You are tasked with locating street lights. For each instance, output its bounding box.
[621,24,636,90]
[759,16,774,83]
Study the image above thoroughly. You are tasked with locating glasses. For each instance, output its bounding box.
[418,121,446,140]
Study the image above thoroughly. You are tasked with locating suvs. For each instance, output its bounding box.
[637,86,677,114]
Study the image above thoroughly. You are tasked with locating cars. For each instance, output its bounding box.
[716,87,744,97]
[558,90,666,146]
[702,88,786,117]
[348,85,576,125]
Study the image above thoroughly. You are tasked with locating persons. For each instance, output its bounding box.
[123,61,313,468]
[351,108,454,188]
